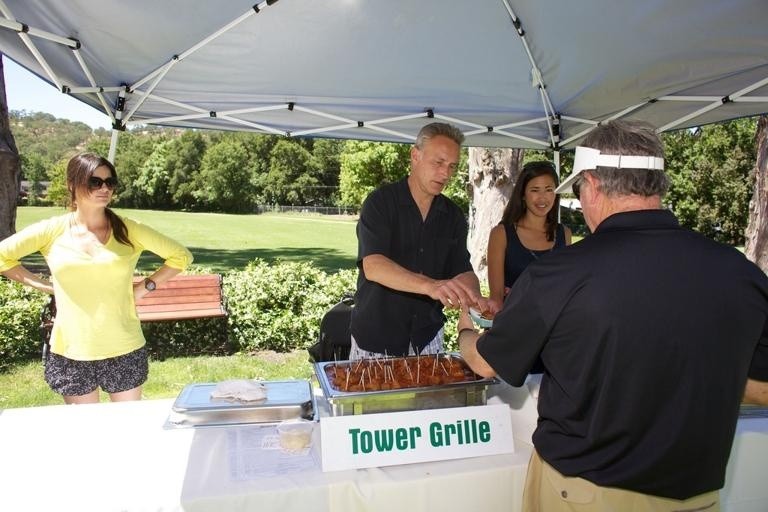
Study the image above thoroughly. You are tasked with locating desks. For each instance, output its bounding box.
[0,372,540,510]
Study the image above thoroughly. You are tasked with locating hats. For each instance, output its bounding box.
[553,146,665,194]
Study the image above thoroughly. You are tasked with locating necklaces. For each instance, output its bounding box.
[513,220,557,261]
[70,211,109,243]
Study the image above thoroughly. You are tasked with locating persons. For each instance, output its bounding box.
[457,116,768,511]
[486,161,574,325]
[0,153,195,405]
[346,121,503,361]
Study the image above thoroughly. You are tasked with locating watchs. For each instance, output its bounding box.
[143,277,157,293]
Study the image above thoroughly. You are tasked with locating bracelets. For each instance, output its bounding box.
[455,327,475,348]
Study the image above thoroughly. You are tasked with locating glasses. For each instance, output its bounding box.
[524,161,557,170]
[87,176,119,191]
[572,177,584,201]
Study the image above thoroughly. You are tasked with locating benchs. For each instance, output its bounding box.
[38,272,231,369]
